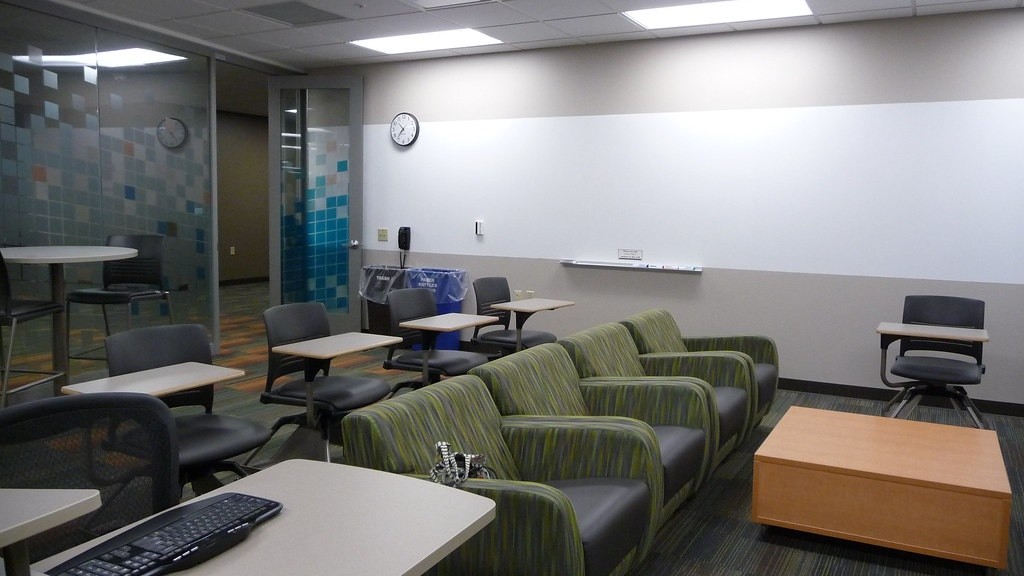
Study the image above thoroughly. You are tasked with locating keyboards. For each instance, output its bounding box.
[44,492,283,576]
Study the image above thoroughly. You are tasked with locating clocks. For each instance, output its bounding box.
[157,116,188,150]
[389,113,419,148]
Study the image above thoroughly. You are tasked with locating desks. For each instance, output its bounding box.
[753,406,1012,570]
[31,457,496,576]
[0,245,139,397]
[398,312,500,381]
[0,487,102,549]
[60,358,246,482]
[271,331,403,421]
[876,322,989,380]
[490,298,574,350]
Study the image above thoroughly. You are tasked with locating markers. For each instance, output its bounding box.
[645,263,703,272]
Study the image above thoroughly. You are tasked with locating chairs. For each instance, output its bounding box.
[0,249,69,407]
[66,234,175,369]
[0,391,181,564]
[383,286,489,400]
[471,276,556,352]
[880,295,989,431]
[82,323,272,531]
[243,303,394,470]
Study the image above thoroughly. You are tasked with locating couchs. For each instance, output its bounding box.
[342,372,659,576]
[554,322,753,485]
[468,342,710,539]
[620,308,779,446]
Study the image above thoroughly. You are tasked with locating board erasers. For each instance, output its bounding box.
[560,258,576,264]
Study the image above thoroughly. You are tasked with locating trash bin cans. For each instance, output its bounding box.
[363,265,405,347]
[406,267,466,350]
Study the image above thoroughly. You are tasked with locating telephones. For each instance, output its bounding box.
[398,226,410,250]
